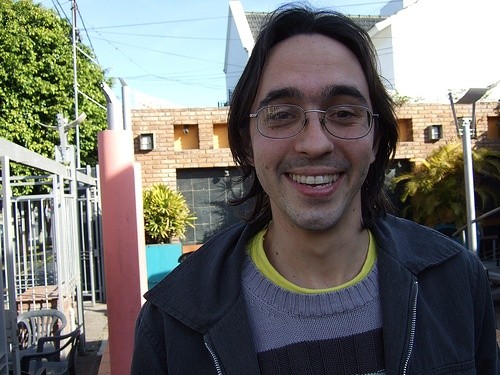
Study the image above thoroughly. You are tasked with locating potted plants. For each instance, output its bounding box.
[144,181,198,291]
[393,139,500,253]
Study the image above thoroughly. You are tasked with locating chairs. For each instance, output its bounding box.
[0,309,80,375]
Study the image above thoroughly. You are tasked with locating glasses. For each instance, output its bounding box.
[250,104,381,140]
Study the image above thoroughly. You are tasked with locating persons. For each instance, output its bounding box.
[129,0,500,375]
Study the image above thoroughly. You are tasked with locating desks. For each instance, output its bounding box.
[16,285,74,362]
